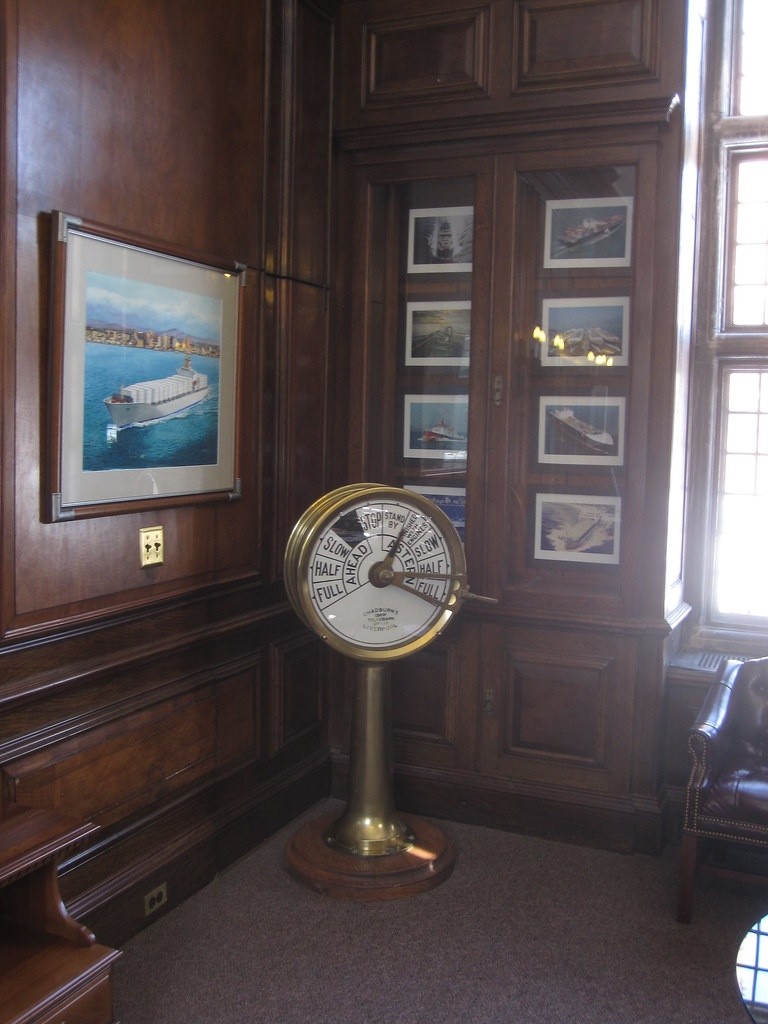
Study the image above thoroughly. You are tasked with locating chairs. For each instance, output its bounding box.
[674,655,768,921]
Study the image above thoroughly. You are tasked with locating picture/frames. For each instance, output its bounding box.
[539,189,633,280]
[395,387,469,468]
[394,476,467,554]
[531,288,631,375]
[42,210,249,524]
[404,200,476,280]
[530,386,627,475]
[526,482,625,572]
[399,291,472,375]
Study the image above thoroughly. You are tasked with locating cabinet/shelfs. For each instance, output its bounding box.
[1,781,126,1024]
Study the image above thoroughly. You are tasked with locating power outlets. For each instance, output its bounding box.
[143,882,169,916]
[140,526,165,569]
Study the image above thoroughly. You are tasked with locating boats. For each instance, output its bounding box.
[102,352,209,428]
[557,214,627,248]
[422,416,465,441]
[559,511,601,550]
[107,424,118,448]
[546,407,614,455]
[436,221,454,261]
